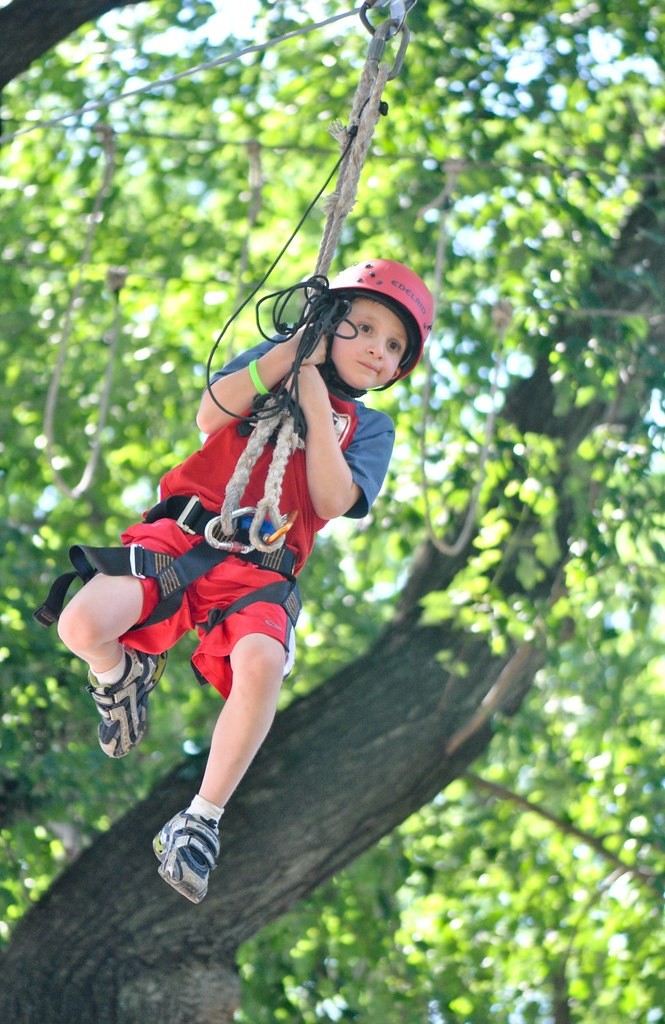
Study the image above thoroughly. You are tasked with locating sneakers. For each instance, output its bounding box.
[151,808,221,905]
[87,641,168,758]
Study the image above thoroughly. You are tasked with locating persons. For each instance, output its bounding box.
[57,259,434,904]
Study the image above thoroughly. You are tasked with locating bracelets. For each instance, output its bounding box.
[249,360,269,396]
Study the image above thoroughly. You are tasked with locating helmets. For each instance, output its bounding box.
[326,256,437,389]
